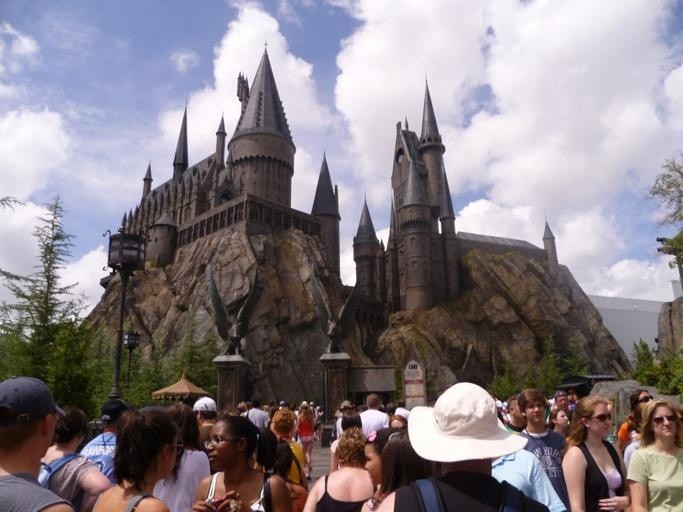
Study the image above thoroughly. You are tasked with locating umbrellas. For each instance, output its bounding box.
[153,368,215,405]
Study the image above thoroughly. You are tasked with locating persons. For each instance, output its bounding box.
[1,375,76,512]
[492,387,682,511]
[40,396,323,511]
[303,382,567,511]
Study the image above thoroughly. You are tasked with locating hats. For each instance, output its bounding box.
[192,396,217,414]
[337,400,355,411]
[0,375,65,427]
[406,382,528,463]
[101,399,129,419]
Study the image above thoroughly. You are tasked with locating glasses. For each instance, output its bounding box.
[637,395,653,403]
[204,435,239,449]
[170,438,185,456]
[653,415,676,423]
[587,412,611,421]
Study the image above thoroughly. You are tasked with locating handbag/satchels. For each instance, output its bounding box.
[36,453,82,490]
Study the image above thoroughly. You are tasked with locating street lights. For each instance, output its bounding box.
[101,229,150,400]
[123,330,139,399]
